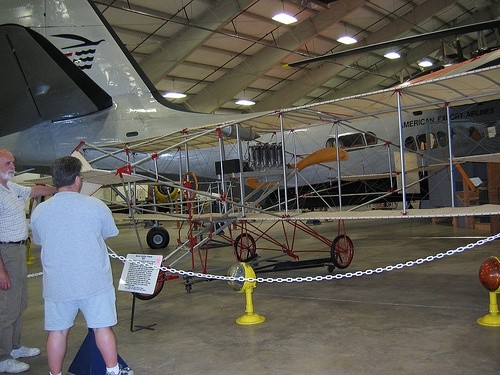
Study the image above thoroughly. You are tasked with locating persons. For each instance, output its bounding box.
[0,148,56,373]
[31,156,135,375]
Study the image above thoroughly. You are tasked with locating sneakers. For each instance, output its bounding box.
[9,346,40,359]
[0,358,30,373]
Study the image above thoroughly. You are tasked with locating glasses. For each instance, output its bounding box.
[79,175,85,179]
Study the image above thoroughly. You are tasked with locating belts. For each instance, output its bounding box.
[0,240,27,244]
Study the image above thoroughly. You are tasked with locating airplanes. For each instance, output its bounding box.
[0,0,500,249]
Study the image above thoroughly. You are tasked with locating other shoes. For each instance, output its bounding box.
[105,364,134,375]
